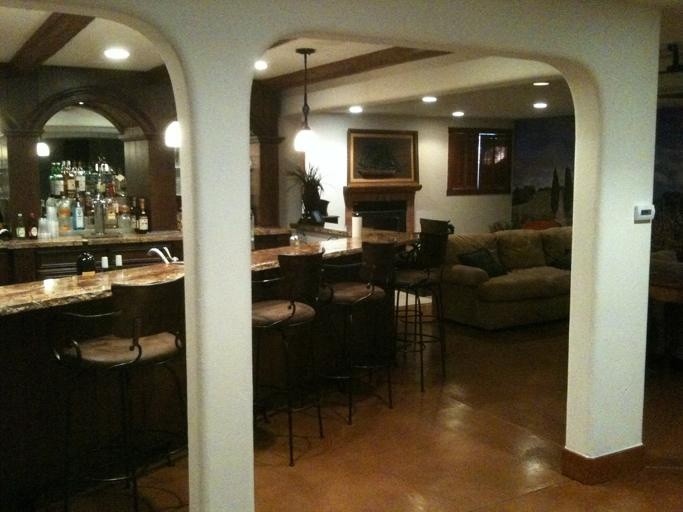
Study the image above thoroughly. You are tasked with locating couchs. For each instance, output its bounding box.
[442,225,571,333]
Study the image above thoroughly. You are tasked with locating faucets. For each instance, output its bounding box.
[147,247,179,264]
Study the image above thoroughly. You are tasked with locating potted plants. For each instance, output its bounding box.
[287,163,329,227]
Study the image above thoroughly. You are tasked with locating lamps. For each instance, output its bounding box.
[294,46,321,155]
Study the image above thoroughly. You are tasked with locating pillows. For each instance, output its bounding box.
[447,225,571,277]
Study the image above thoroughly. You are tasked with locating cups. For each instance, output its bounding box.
[351,217,361,239]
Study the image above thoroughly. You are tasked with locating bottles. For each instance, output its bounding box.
[287,229,299,250]
[100,256,108,272]
[77,240,96,276]
[114,254,122,270]
[13,155,149,239]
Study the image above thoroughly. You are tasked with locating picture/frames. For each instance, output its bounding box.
[347,128,419,185]
